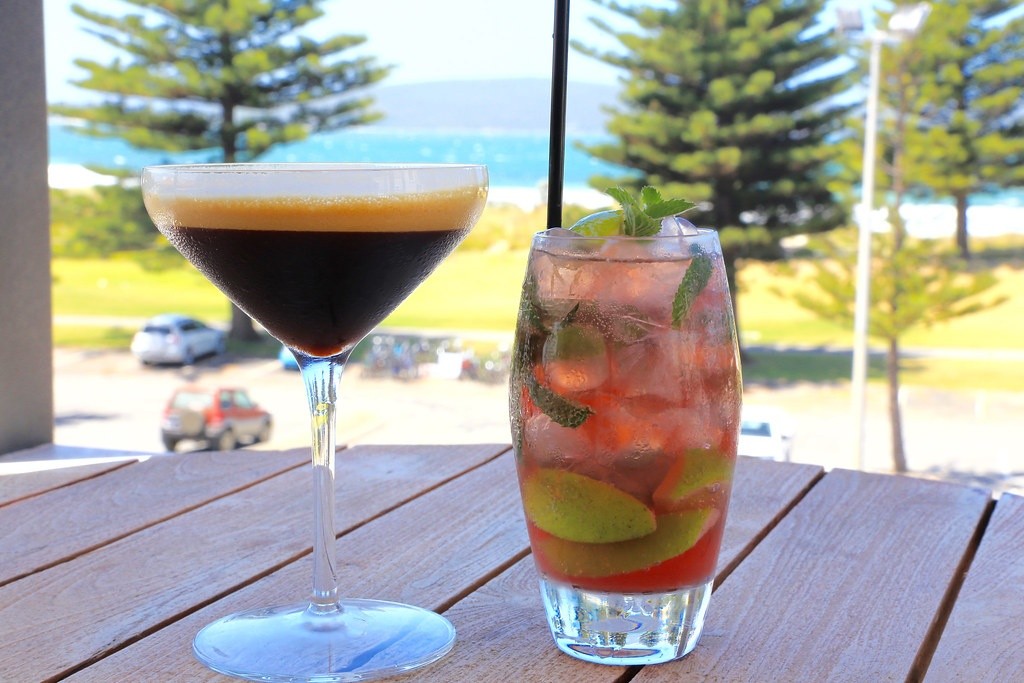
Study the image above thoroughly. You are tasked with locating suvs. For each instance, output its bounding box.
[133,316,221,366]
[161,387,274,451]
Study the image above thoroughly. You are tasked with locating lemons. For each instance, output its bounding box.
[521,447,729,578]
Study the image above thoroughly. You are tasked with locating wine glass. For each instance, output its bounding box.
[139,162,487,683]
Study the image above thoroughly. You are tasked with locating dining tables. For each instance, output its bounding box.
[0,445,1022,683]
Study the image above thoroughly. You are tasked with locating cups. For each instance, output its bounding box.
[506,228,744,667]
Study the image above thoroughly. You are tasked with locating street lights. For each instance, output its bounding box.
[834,5,926,471]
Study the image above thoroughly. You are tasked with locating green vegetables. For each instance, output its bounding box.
[508,185,716,451]
